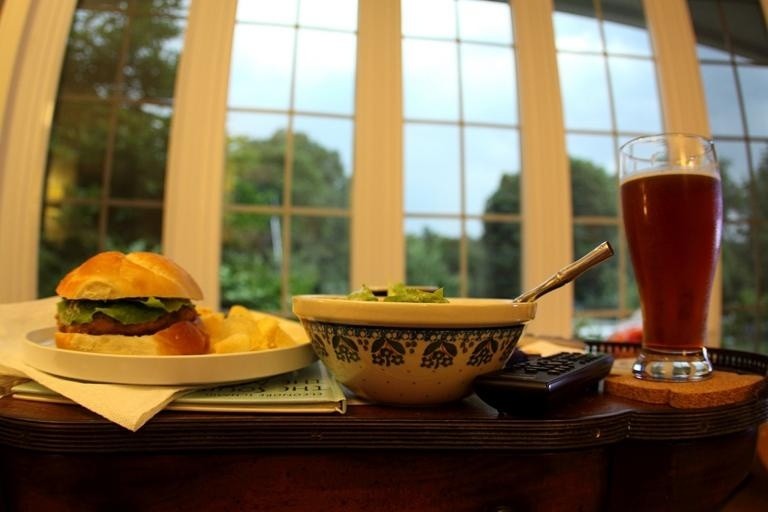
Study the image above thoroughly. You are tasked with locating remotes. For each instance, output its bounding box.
[473,347,616,412]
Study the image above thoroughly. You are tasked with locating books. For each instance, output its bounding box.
[10,381,348,416]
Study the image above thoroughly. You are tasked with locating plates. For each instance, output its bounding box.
[20,305,320,388]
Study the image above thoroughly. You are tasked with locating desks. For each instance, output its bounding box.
[1,378,768,511]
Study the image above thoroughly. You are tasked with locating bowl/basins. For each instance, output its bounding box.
[294,287,540,408]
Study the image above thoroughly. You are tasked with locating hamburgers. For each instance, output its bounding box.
[55,250,210,356]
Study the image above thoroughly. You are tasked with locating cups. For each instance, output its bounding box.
[615,129,728,383]
